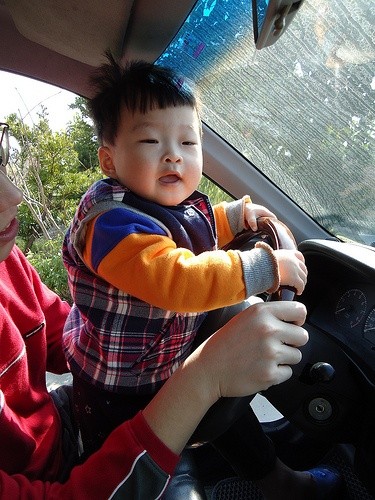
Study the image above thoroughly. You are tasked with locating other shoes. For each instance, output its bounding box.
[307,463,343,496]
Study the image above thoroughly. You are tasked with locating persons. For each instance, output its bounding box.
[0,145,308,500]
[61,44,308,500]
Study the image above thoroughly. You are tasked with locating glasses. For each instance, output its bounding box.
[0,122,10,171]
